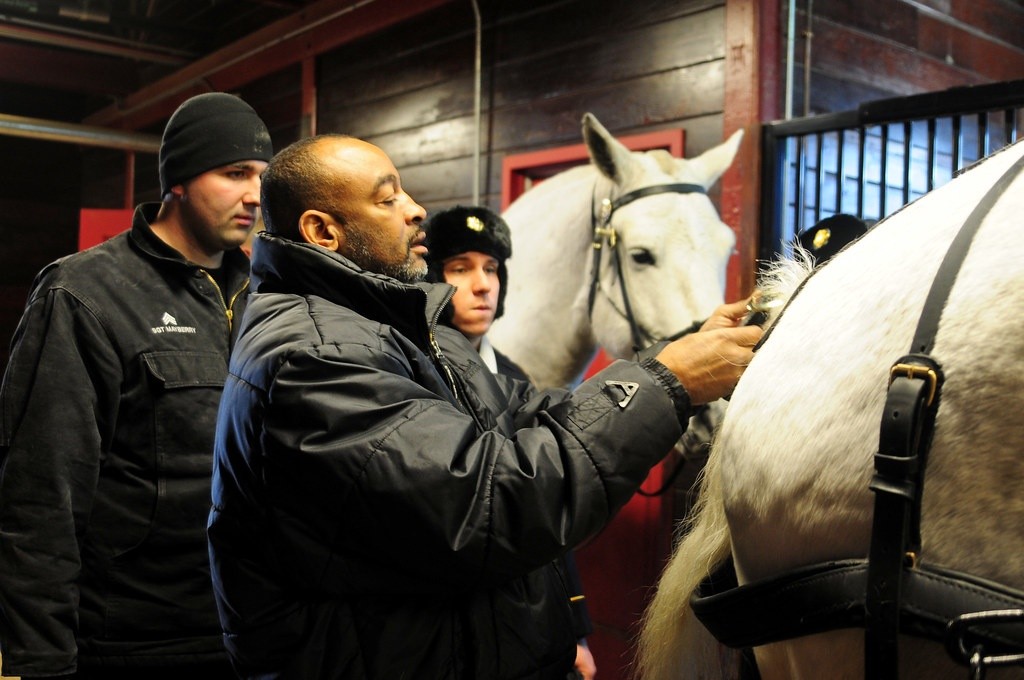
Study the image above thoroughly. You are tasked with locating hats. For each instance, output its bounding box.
[795,213,867,267]
[423,206,511,319]
[159,92,273,199]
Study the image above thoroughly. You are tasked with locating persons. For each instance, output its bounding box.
[12,89,272,678]
[413,205,598,679]
[205,133,772,679]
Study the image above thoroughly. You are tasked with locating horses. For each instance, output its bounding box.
[618,138,1024,680]
[484,113,744,461]
